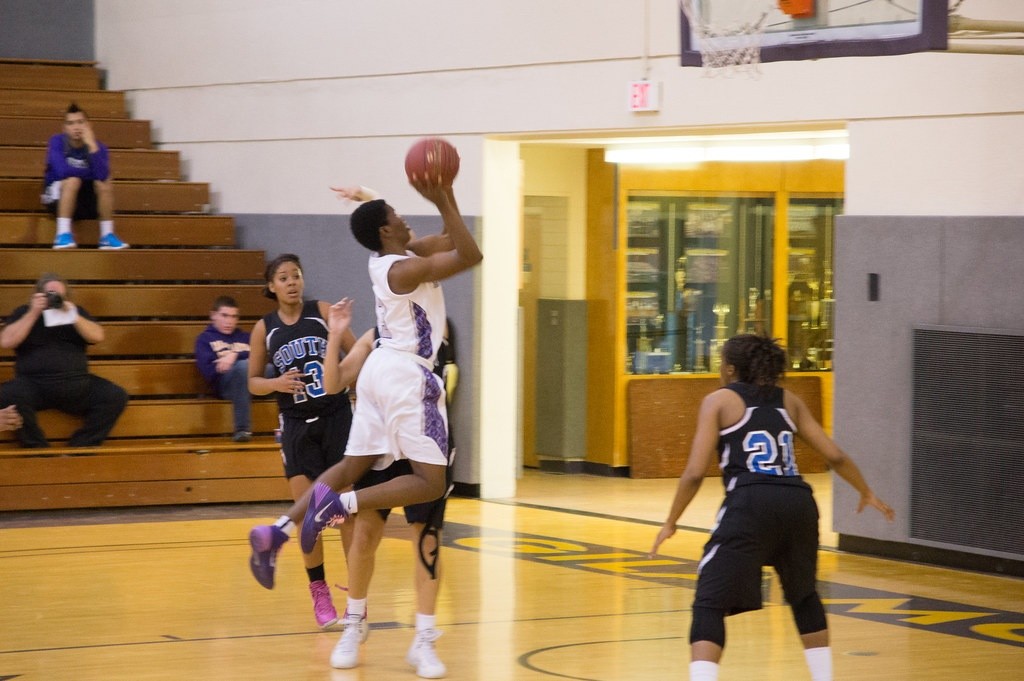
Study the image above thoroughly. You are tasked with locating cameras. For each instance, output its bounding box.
[42,291,63,309]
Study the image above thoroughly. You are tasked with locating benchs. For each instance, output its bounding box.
[0,56,356,508]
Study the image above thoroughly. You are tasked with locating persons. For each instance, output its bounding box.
[248,172,482,589]
[194,295,283,442]
[0,274,129,458]
[39,102,130,250]
[649,334,895,681]
[247,254,359,625]
[322,298,460,679]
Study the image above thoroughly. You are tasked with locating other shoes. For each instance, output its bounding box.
[277,432,282,442]
[235,430,251,441]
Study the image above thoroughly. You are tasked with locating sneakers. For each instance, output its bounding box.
[301,481,348,554]
[53,232,75,249]
[250,525,289,589]
[309,580,337,628]
[408,626,446,678]
[99,233,129,249]
[330,616,369,669]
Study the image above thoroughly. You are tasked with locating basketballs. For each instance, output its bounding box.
[404,137,461,184]
